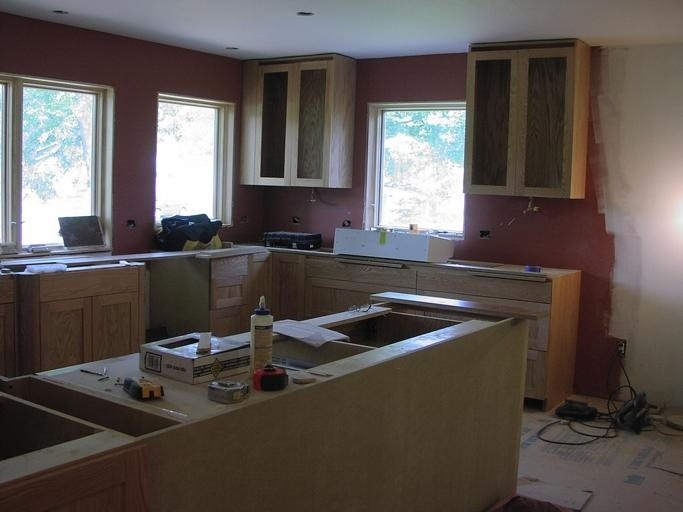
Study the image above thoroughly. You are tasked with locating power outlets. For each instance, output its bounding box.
[617,339,626,358]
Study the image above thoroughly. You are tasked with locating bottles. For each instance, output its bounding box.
[249,295,275,381]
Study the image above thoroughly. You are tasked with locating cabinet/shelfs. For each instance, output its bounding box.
[464,38,591,199]
[19,265,145,375]
[239,53,356,189]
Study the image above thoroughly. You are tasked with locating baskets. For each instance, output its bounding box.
[263,232,321,250]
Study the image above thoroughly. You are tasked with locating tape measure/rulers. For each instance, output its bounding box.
[208,364,290,404]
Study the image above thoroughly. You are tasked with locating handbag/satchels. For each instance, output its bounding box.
[155,214,222,251]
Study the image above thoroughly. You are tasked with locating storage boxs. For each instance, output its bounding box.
[140,332,250,386]
[333,228,454,263]
